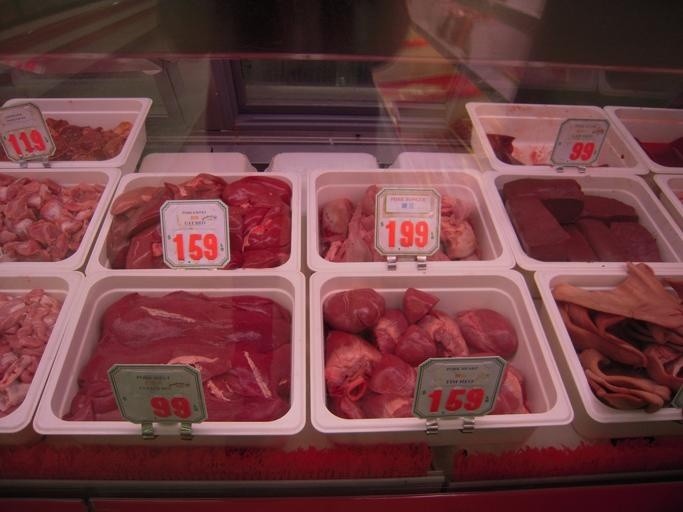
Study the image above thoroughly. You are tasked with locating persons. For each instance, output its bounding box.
[151,1,409,161]
